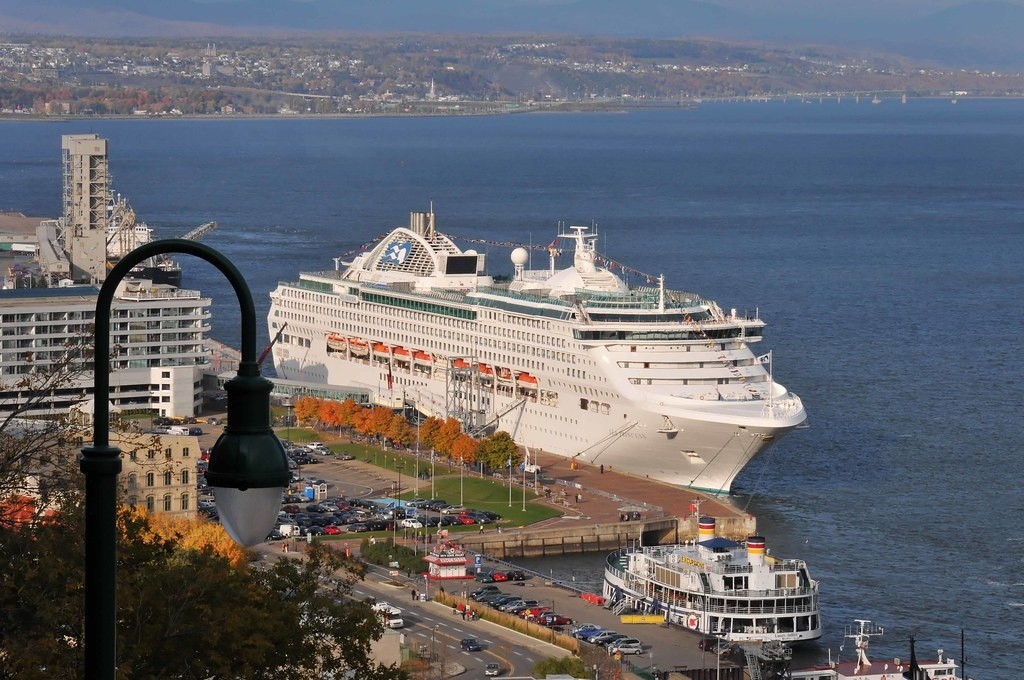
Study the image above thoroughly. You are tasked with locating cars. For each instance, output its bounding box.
[197,437,503,541]
[698,636,739,656]
[460,638,483,653]
[485,662,501,677]
[153,416,224,436]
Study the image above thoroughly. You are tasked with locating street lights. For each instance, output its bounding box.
[76,237,294,679]
[603,495,822,642]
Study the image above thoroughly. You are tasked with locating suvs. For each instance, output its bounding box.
[470,584,643,656]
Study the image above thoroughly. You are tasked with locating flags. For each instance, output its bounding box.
[337,231,769,399]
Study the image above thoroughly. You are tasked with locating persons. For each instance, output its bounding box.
[575,492,582,503]
[282,541,290,553]
[370,535,375,544]
[412,589,420,600]
[402,528,432,544]
[479,522,484,534]
[452,603,478,621]
[495,524,501,534]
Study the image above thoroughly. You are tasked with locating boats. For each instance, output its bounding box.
[105,193,183,285]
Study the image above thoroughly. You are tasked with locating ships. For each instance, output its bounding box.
[266,197,807,539]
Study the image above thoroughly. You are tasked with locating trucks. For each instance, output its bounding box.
[372,601,405,629]
